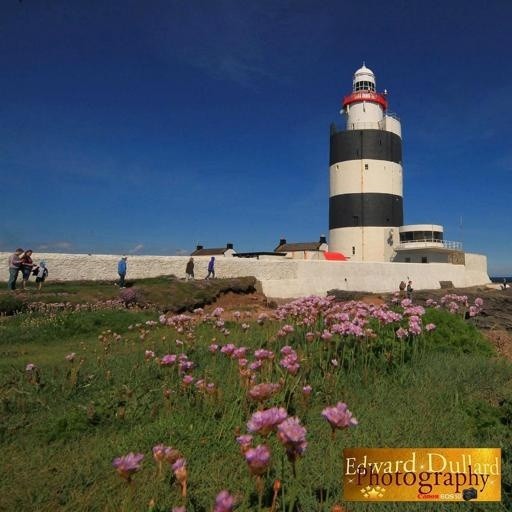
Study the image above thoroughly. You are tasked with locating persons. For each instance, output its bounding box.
[185,257,195,282]
[205,257,216,280]
[503,278,506,288]
[398,281,406,302]
[8,248,25,291]
[406,281,413,301]
[20,249,38,291]
[117,256,128,289]
[31,259,49,290]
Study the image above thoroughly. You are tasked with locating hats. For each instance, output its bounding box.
[121,256,127,258]
